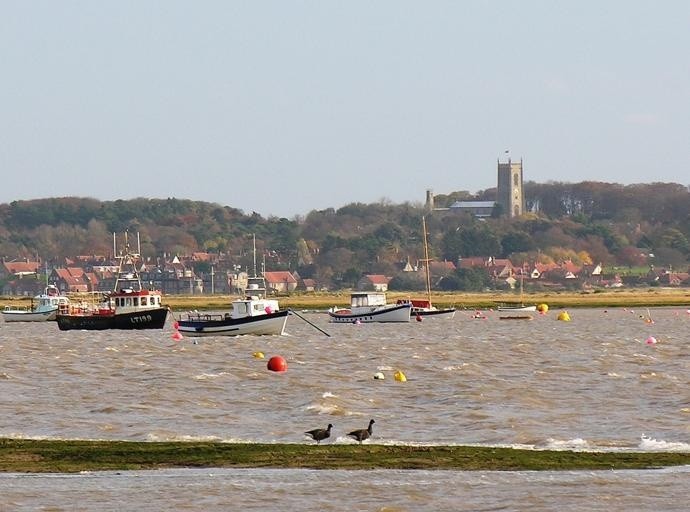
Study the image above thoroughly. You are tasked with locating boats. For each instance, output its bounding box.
[329,291,457,323]
[177,296,289,338]
[0,285,170,331]
[498,305,537,313]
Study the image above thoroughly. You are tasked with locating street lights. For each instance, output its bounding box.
[209,261,218,295]
[260,262,264,277]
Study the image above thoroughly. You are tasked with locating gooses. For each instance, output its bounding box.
[346,419,376,445]
[303,422,333,444]
